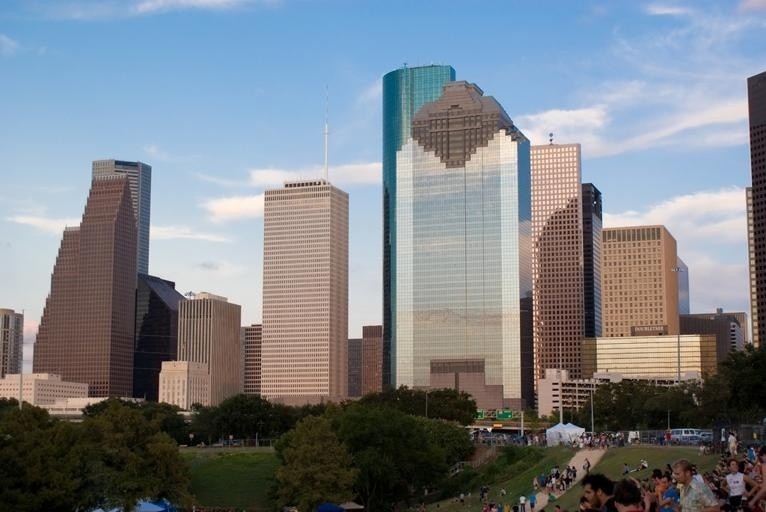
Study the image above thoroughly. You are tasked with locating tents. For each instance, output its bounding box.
[546,422,585,447]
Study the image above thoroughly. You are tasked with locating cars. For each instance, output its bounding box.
[663,428,713,446]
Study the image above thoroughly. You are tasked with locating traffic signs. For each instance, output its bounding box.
[471,409,484,420]
[495,410,512,420]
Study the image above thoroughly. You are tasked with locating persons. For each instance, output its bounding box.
[580,458,722,510]
[480,486,562,511]
[455,487,471,506]
[664,431,673,449]
[482,435,486,443]
[533,457,590,494]
[511,431,547,446]
[399,479,440,510]
[566,431,641,452]
[699,431,765,511]
